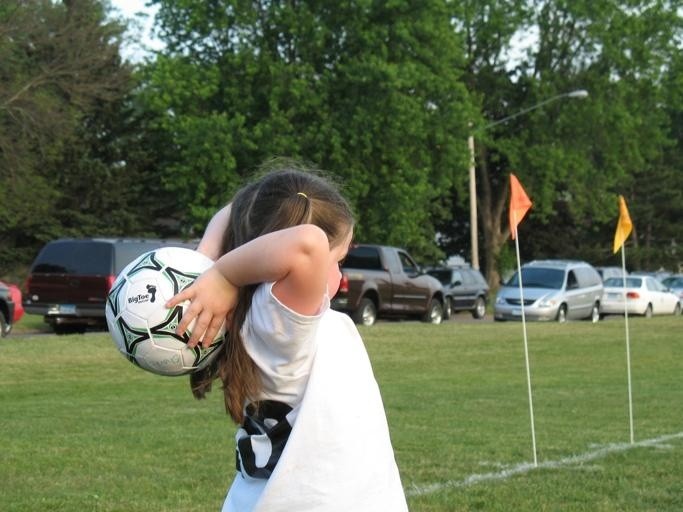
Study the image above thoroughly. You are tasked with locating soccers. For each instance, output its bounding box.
[106,247,226,377]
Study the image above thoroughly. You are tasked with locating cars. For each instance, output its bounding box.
[494,259,603,323]
[596,266,683,318]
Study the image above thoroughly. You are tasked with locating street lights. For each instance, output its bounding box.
[468,89,588,269]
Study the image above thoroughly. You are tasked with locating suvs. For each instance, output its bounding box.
[23,240,198,334]
[428,265,490,320]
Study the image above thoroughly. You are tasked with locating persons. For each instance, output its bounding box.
[161,164,410,512]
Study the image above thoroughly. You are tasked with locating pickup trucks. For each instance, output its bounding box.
[330,243,445,324]
